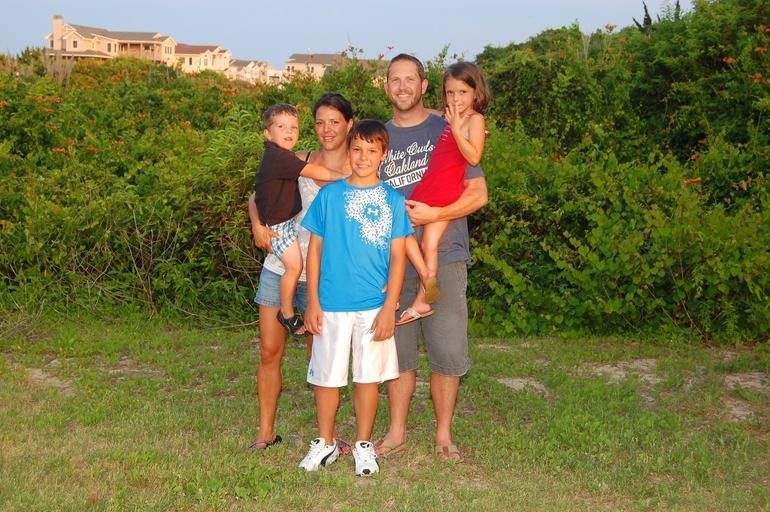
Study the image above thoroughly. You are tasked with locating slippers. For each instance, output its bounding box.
[336,438,351,454]
[375,438,407,457]
[248,434,282,451]
[423,277,440,304]
[394,307,435,326]
[434,444,464,464]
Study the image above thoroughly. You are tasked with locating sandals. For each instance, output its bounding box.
[277,310,308,338]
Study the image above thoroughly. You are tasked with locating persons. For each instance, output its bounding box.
[394,62,490,325]
[249,92,355,457]
[301,120,416,475]
[371,54,492,465]
[253,103,350,337]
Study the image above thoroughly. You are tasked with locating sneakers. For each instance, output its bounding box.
[352,440,380,477]
[298,437,339,472]
